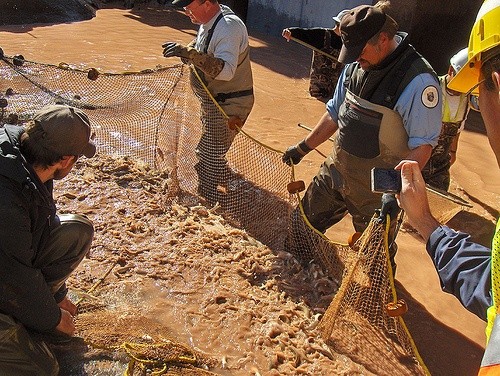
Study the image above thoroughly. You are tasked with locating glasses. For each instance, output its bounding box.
[464,79,487,113]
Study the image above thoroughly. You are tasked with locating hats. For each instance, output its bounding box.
[332,10,350,25]
[338,5,387,64]
[171,0,194,7]
[32,103,96,159]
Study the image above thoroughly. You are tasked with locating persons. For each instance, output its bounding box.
[393,0,500,376]
[282,10,351,99]
[0,104,96,376]
[282,1,443,297]
[421,47,469,192]
[162,0,254,199]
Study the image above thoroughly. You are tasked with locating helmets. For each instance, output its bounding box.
[450,48,468,73]
[446,2,500,97]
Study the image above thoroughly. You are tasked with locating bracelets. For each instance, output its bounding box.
[450,151,456,155]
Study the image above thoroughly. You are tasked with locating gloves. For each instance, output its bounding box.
[162,41,225,80]
[281,146,304,167]
[374,193,400,226]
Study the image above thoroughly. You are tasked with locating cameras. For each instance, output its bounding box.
[371,167,402,194]
